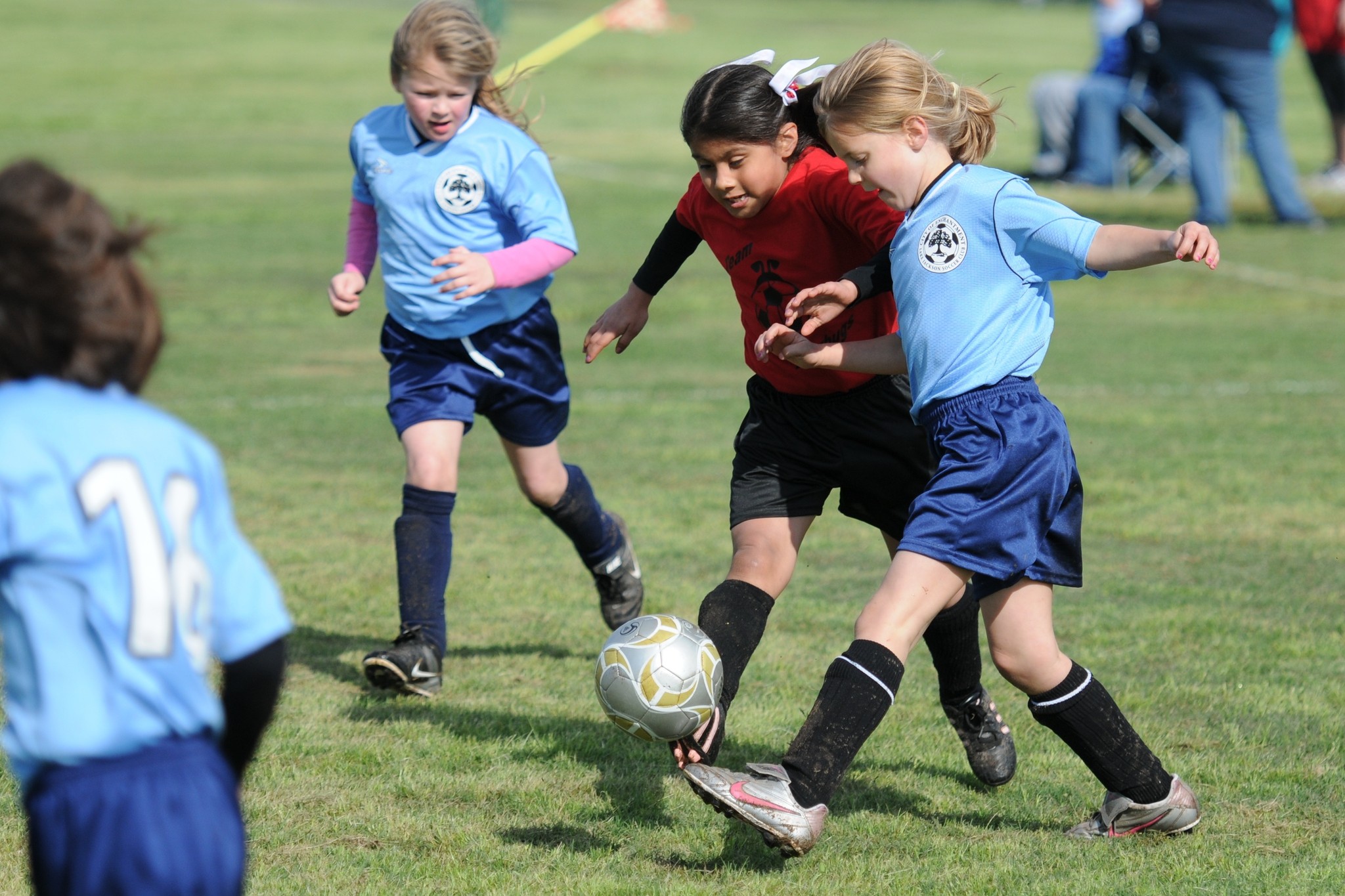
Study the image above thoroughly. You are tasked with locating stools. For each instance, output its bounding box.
[1116,96,1194,200]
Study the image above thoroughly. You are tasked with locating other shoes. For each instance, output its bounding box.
[1026,153,1071,179]
[1192,203,1230,224]
[1315,161,1345,193]
[1070,164,1124,189]
[1276,205,1314,228]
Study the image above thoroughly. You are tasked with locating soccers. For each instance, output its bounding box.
[593,613,726,743]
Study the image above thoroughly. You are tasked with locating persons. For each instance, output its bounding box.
[0,152,298,896]
[670,39,1223,861]
[1028,1,1343,233]
[579,50,1025,786]
[324,7,641,701]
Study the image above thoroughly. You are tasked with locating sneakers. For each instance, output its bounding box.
[590,512,644,628]
[680,756,826,859]
[1064,772,1203,839]
[361,632,446,702]
[668,702,724,772]
[941,686,1020,788]
